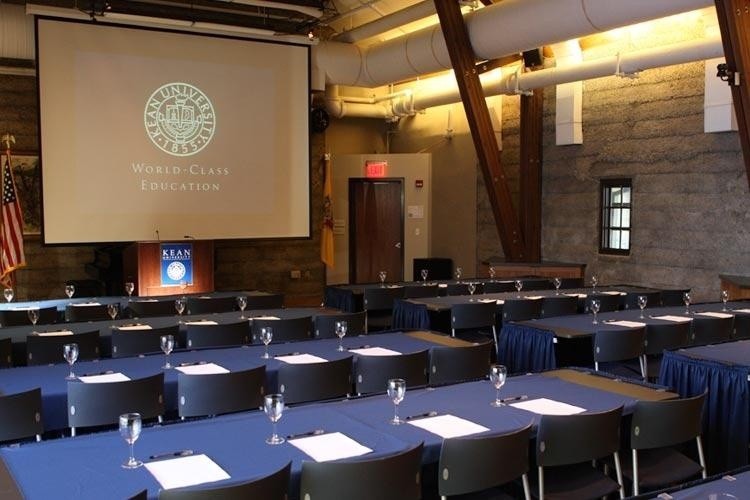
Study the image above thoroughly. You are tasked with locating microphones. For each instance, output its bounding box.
[184,236,195,240]
[156,230,159,240]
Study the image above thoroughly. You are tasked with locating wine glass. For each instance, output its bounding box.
[386,379,407,427]
[262,394,286,445]
[1,268,734,380]
[118,413,144,469]
[488,364,507,410]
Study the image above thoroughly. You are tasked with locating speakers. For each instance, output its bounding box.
[66,278,106,297]
[523,48,543,67]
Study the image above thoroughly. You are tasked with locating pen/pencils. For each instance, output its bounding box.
[606,320,619,322]
[406,411,438,420]
[275,352,300,357]
[44,329,70,333]
[179,360,211,367]
[287,430,325,438]
[388,283,401,287]
[347,345,370,350]
[149,448,195,461]
[501,395,529,403]
[121,324,143,327]
[84,370,115,376]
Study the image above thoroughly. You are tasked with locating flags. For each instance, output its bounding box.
[0,149,30,291]
